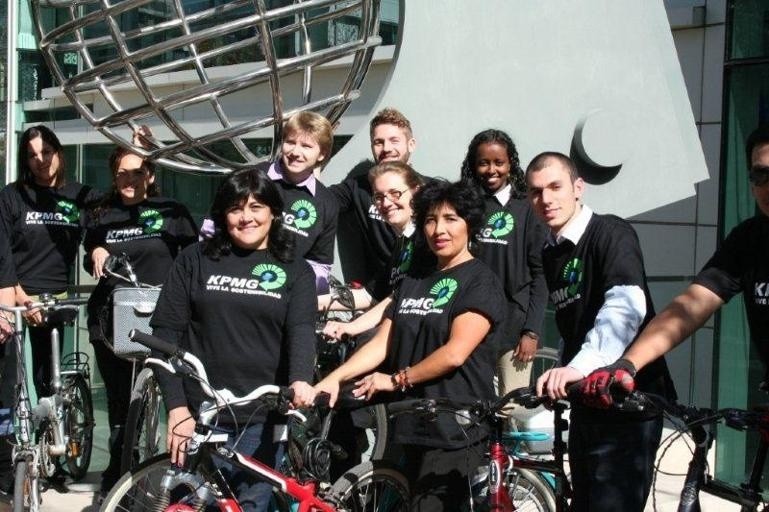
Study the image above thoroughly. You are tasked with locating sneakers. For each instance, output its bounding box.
[98,466,137,508]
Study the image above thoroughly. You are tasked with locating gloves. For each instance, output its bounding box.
[568,358,638,411]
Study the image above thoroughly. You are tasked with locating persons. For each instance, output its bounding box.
[583,125,768,406]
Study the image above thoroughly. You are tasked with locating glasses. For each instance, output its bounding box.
[371,186,412,205]
[748,164,768,186]
